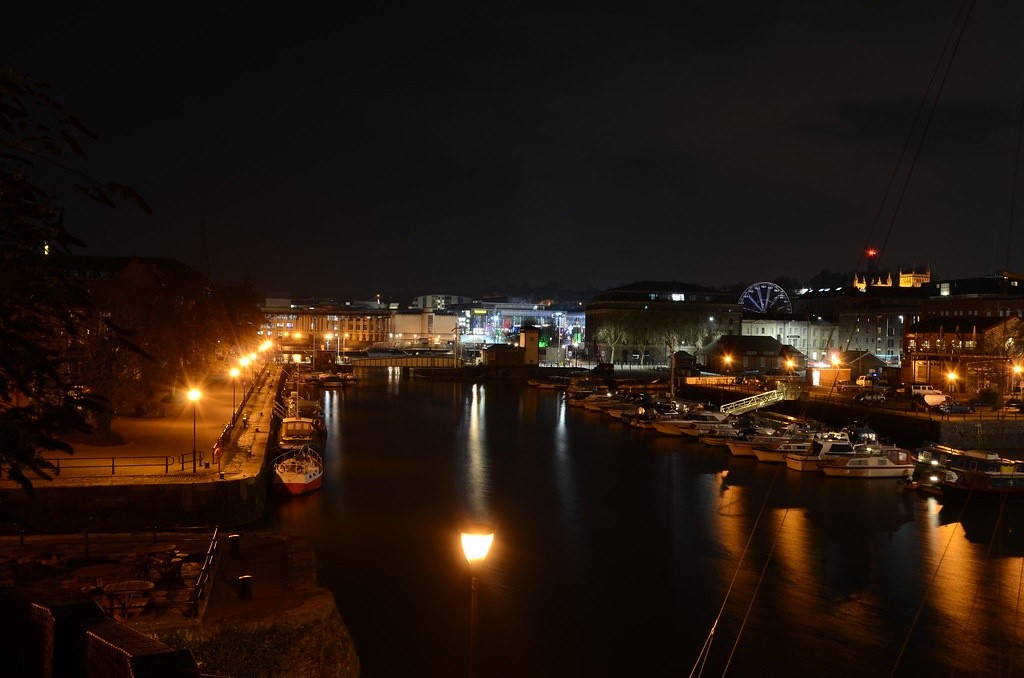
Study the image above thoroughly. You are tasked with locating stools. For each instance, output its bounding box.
[153,553,189,607]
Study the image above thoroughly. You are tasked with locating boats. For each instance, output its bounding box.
[269,362,359,495]
[528,376,1024,506]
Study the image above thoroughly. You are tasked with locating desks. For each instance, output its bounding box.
[73,541,180,613]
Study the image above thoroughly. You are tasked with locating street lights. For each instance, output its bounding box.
[240,356,251,405]
[456,522,496,678]
[229,367,239,427]
[183,385,206,473]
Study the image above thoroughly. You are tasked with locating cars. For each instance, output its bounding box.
[837,380,862,392]
[856,376,874,387]
[935,402,976,414]
[881,387,894,395]
[893,384,905,393]
[853,390,886,405]
[1007,406,1020,413]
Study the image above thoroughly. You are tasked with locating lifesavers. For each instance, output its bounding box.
[301,444,309,454]
[277,465,285,473]
[293,466,302,474]
[313,417,325,430]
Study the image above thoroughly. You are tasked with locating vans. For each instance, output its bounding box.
[911,385,942,397]
